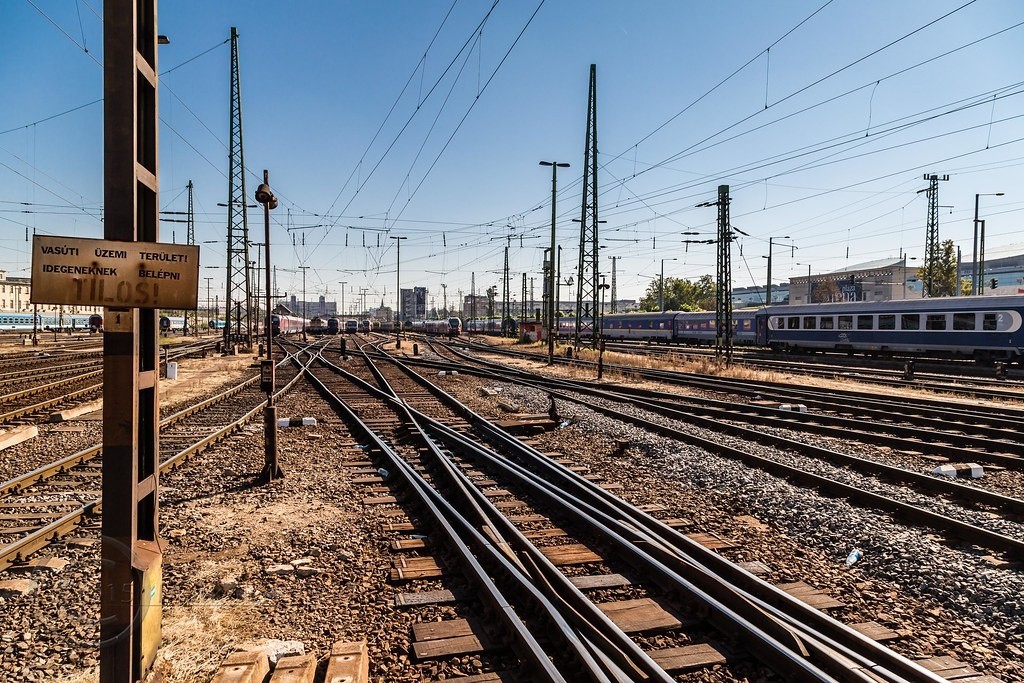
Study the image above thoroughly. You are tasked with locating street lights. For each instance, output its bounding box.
[972,193,1004,295]
[204,278,213,335]
[340,281,347,330]
[298,266,310,342]
[390,236,408,349]
[762,236,790,306]
[254,169,285,484]
[359,294,365,315]
[441,284,447,318]
[660,258,677,311]
[973,220,985,296]
[796,263,810,304]
[362,288,369,312]
[357,298,362,315]
[539,161,570,364]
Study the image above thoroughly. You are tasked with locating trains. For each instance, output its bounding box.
[89,314,104,333]
[326,317,345,333]
[208,320,263,328]
[466,317,517,334]
[346,319,358,333]
[310,316,328,332]
[359,320,413,332]
[0,315,91,332]
[412,317,462,336]
[264,315,311,336]
[159,317,202,331]
[552,294,1024,359]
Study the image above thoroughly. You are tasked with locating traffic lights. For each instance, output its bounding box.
[991,278,998,289]
